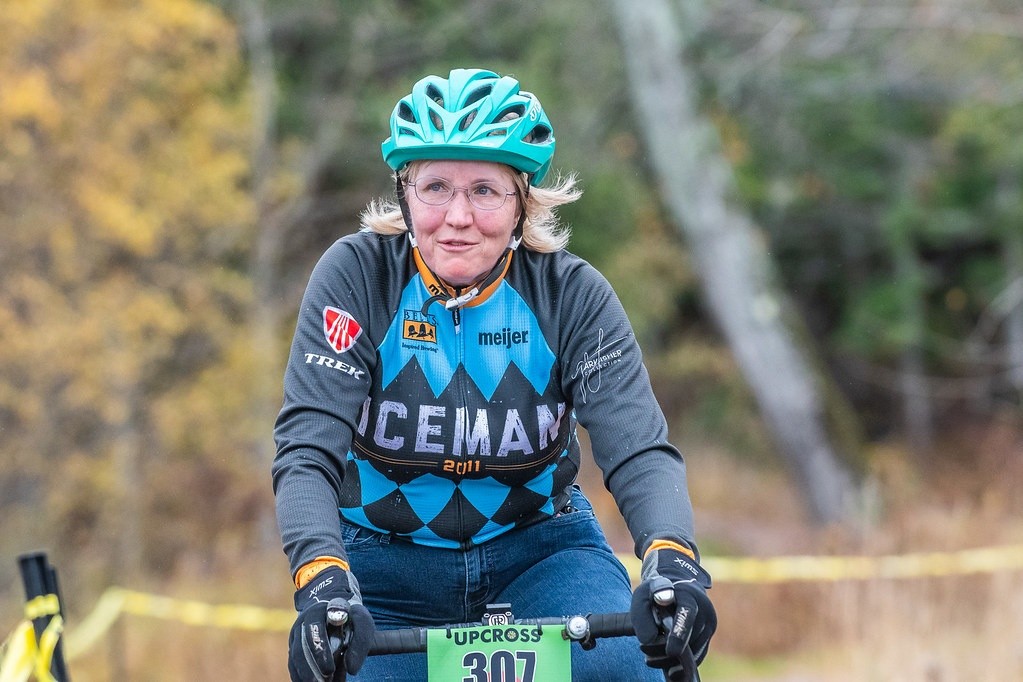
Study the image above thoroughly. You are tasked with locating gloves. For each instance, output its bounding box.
[287,565,375,682]
[629,548,717,681]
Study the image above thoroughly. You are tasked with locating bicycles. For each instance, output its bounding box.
[326,577,701,682]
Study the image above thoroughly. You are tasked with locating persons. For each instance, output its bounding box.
[268,69,717,682]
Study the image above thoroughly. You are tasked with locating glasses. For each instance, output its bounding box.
[401,176,519,210]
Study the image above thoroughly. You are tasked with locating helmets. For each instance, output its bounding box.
[380,68,555,186]
